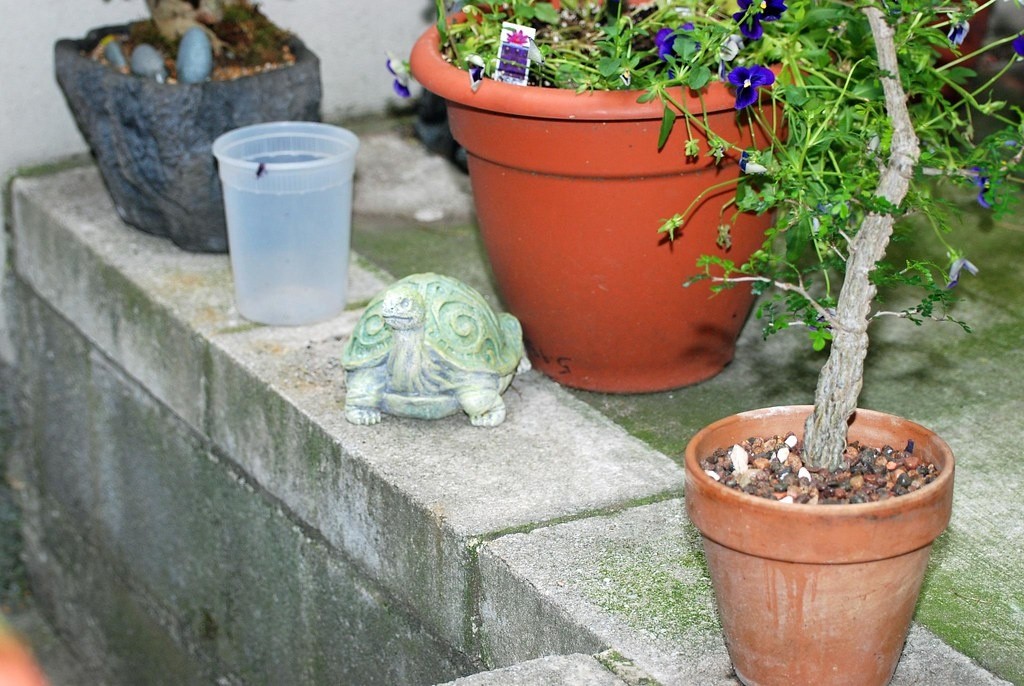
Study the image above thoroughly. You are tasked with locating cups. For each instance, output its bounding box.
[210,120,360,329]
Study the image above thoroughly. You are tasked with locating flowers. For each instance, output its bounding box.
[387,0,1024,331]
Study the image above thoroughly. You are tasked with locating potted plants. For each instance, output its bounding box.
[54,1,324,256]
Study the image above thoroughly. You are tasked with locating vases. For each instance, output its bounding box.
[411,0,833,394]
[685,404,953,686]
